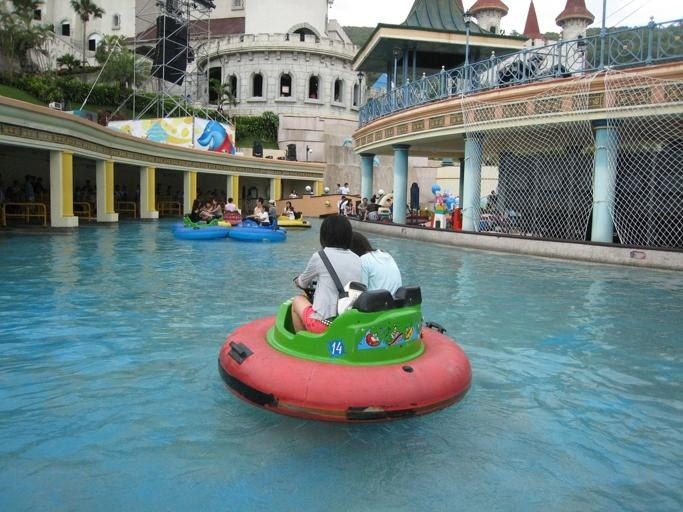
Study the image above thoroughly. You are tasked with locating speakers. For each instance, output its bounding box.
[151,15,190,86]
[253,140,263,158]
[287,144,296,161]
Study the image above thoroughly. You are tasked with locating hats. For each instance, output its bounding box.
[268,200,276,204]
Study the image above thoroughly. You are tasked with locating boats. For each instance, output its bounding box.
[217,280,472,425]
[173,211,311,243]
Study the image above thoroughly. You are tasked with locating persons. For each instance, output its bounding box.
[290,212,361,334]
[74,179,97,216]
[282,201,296,220]
[386,198,393,220]
[167,185,171,196]
[349,231,403,298]
[134,185,139,203]
[156,182,161,197]
[114,184,130,212]
[1,174,46,224]
[487,190,497,208]
[191,188,237,224]
[336,183,385,222]
[250,197,277,227]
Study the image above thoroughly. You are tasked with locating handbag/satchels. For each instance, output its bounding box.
[336,282,367,315]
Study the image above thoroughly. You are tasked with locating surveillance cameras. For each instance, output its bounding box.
[463,16,477,24]
[309,149,313,153]
